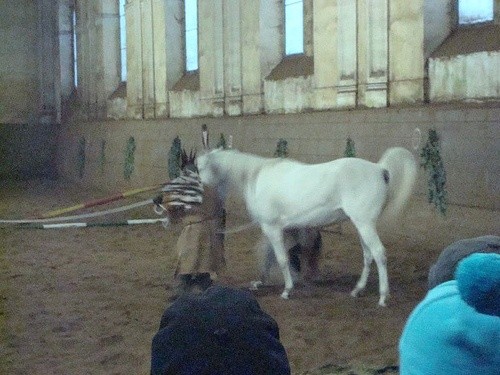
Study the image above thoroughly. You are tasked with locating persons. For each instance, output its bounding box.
[148,284,291,375]
[282,226,325,299]
[161,162,228,301]
[400,233,500,375]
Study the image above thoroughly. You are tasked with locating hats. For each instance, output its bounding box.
[426,234,500,295]
[396,252,500,375]
[149,287,290,375]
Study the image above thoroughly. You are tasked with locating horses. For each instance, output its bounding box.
[161,145,421,308]
